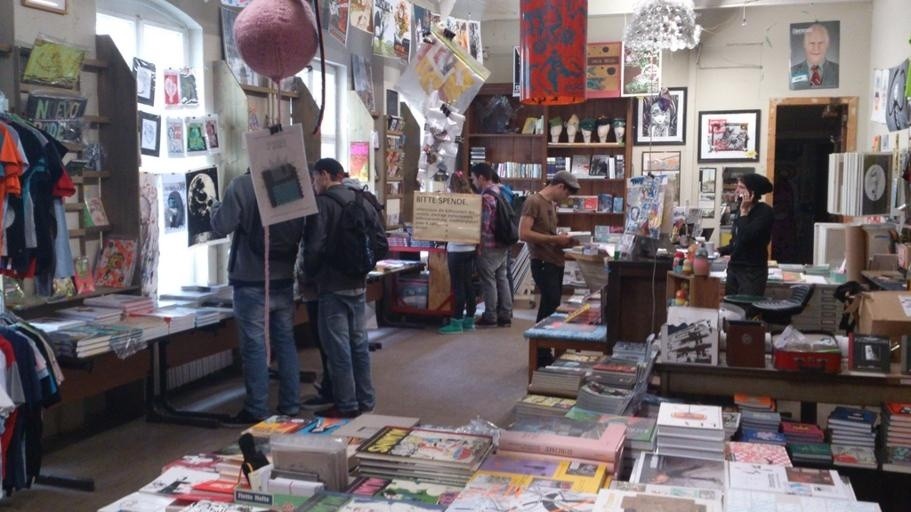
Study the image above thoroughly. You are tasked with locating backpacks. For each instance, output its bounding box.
[482,189,518,245]
[319,188,380,277]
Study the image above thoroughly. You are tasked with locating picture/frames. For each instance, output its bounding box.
[721,164,757,230]
[696,108,762,163]
[629,86,688,148]
[640,151,681,207]
[787,19,841,93]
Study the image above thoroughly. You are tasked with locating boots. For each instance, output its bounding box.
[438,319,463,333]
[461,318,475,330]
[454,327,455,328]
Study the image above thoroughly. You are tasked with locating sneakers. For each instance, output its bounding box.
[358,403,376,414]
[499,320,512,327]
[216,411,262,428]
[314,407,360,419]
[301,398,334,411]
[475,317,498,328]
[271,408,295,418]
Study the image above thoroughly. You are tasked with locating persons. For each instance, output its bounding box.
[475,163,514,327]
[519,171,582,369]
[168,191,184,228]
[292,171,362,412]
[210,168,306,428]
[713,175,775,297]
[492,170,514,316]
[299,158,389,423]
[438,170,477,335]
[791,24,840,90]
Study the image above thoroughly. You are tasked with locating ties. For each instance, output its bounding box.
[810,66,821,86]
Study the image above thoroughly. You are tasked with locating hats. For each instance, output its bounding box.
[737,174,773,197]
[554,170,581,189]
[593,112,611,124]
[580,116,596,130]
[549,115,563,126]
[564,113,580,129]
[611,113,626,127]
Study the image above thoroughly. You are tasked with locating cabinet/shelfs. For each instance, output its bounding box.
[1,34,152,314]
[380,114,409,225]
[449,76,635,304]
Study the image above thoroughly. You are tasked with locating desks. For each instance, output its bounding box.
[526,290,609,390]
[522,273,911,479]
[26,254,429,492]
[93,410,881,512]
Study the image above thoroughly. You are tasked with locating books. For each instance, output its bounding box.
[567,231,591,244]
[546,155,624,179]
[769,260,830,285]
[20,294,221,360]
[557,288,603,325]
[374,222,435,269]
[470,146,487,168]
[486,161,542,178]
[828,151,893,217]
[159,283,234,321]
[665,305,718,367]
[881,400,911,464]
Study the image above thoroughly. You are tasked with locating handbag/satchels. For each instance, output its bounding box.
[751,228,770,246]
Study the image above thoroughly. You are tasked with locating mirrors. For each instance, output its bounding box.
[695,166,717,221]
